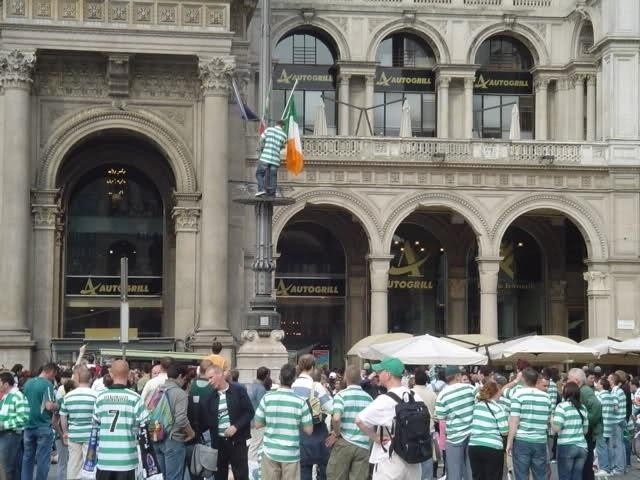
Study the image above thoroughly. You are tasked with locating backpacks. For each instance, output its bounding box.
[147,384,179,443]
[188,379,213,434]
[305,382,322,426]
[380,389,432,464]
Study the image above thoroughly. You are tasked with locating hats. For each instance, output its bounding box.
[445,365,464,378]
[371,358,404,377]
[329,372,337,379]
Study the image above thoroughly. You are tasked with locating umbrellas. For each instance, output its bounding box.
[346,334,640,374]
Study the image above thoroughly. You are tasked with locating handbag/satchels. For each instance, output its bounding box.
[484,400,507,452]
[310,382,323,424]
[190,443,218,478]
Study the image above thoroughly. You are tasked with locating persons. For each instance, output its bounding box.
[550,382,589,479]
[254,364,314,480]
[21,363,60,480]
[586,372,596,386]
[506,367,552,480]
[194,365,255,480]
[255,121,287,196]
[468,382,509,480]
[434,366,477,480]
[202,342,228,370]
[91,366,108,390]
[128,367,150,394]
[516,359,530,372]
[267,377,272,390]
[499,371,533,480]
[230,370,247,393]
[250,367,267,480]
[360,369,380,400]
[188,360,213,443]
[461,374,469,384]
[11,364,24,376]
[60,371,98,480]
[55,373,72,400]
[534,374,550,392]
[308,354,352,396]
[550,381,588,480]
[82,360,161,480]
[609,371,640,474]
[59,343,99,368]
[142,357,175,403]
[410,371,438,478]
[541,368,558,435]
[147,361,195,480]
[432,368,449,478]
[99,375,114,390]
[0,372,30,480]
[567,368,604,480]
[583,362,639,480]
[152,365,162,378]
[354,357,435,480]
[595,379,614,478]
[58,380,80,480]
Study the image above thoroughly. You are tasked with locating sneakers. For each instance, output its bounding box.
[255,190,266,196]
[594,470,607,477]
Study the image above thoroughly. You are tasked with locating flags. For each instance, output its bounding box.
[259,75,272,139]
[232,78,262,122]
[281,87,288,113]
[280,80,303,176]
